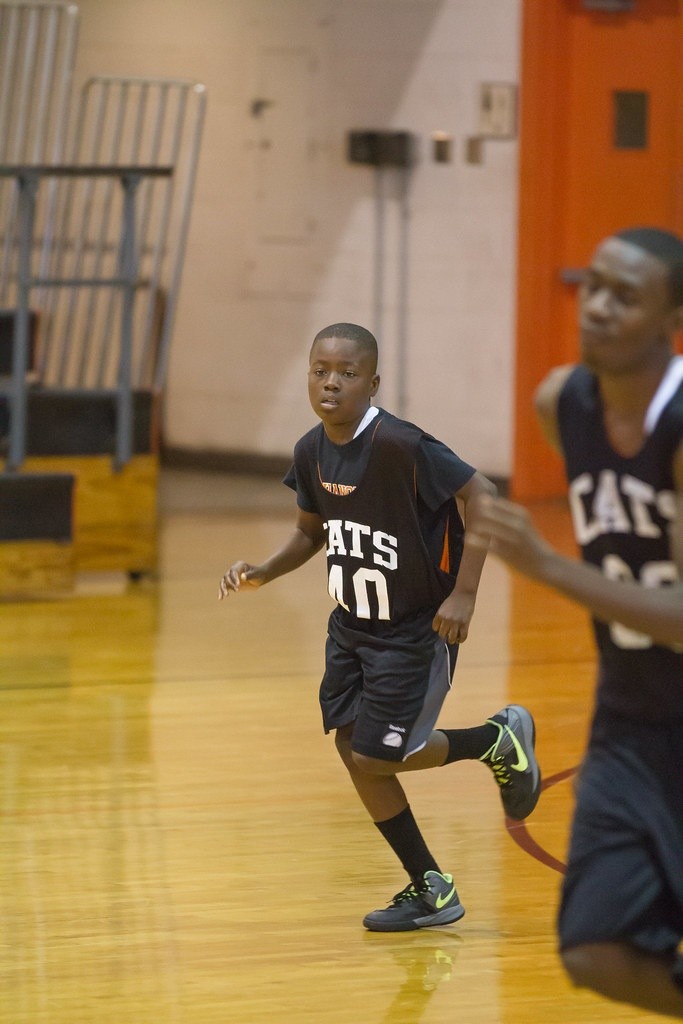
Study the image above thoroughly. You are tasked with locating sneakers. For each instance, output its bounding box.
[363,869,466,932]
[478,704,542,821]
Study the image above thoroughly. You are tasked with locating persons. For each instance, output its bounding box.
[217,323,539,931]
[458,223,683,1019]
